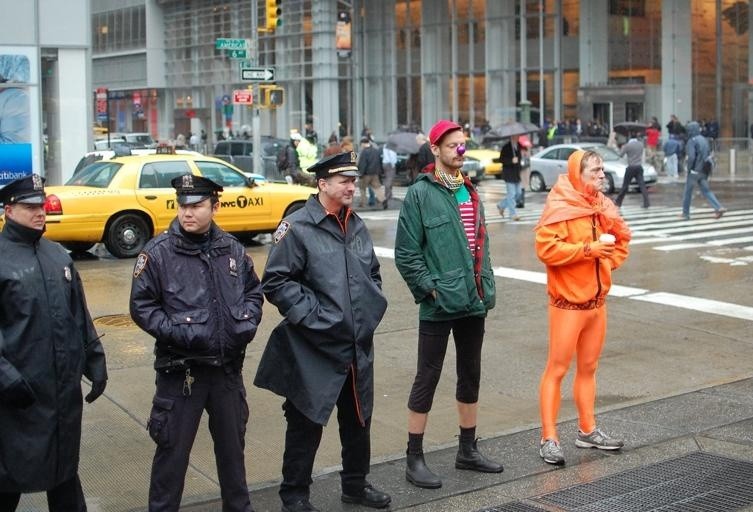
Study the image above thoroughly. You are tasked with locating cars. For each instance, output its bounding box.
[369,134,508,187]
[527,142,658,192]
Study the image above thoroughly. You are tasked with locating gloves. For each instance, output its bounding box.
[83,378,109,405]
[1,373,39,411]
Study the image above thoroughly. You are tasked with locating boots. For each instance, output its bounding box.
[453,434,504,474]
[405,452,444,489]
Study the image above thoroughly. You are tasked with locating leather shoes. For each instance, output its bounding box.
[339,479,392,509]
[278,495,326,512]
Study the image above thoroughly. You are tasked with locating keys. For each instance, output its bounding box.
[182,364,194,396]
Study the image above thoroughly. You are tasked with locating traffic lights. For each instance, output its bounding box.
[266,89,283,107]
[266,0,282,28]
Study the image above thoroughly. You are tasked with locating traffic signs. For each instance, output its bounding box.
[226,50,247,59]
[214,38,246,51]
[241,69,274,81]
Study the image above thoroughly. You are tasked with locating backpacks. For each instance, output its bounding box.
[275,143,293,170]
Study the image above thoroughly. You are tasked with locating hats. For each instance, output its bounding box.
[0,172,48,207]
[427,118,465,147]
[306,151,364,180]
[171,173,224,206]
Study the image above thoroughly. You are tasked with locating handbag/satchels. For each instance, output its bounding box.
[702,155,718,176]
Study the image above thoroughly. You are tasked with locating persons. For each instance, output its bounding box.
[533,151,628,465]
[0,171,110,512]
[395,120,504,489]
[252,148,392,512]
[129,175,264,512]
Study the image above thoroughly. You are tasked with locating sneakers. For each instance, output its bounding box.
[574,426,625,451]
[357,197,390,211]
[538,435,568,466]
[496,203,522,222]
[713,207,727,219]
[675,212,689,222]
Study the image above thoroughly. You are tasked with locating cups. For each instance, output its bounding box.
[598,233,617,246]
